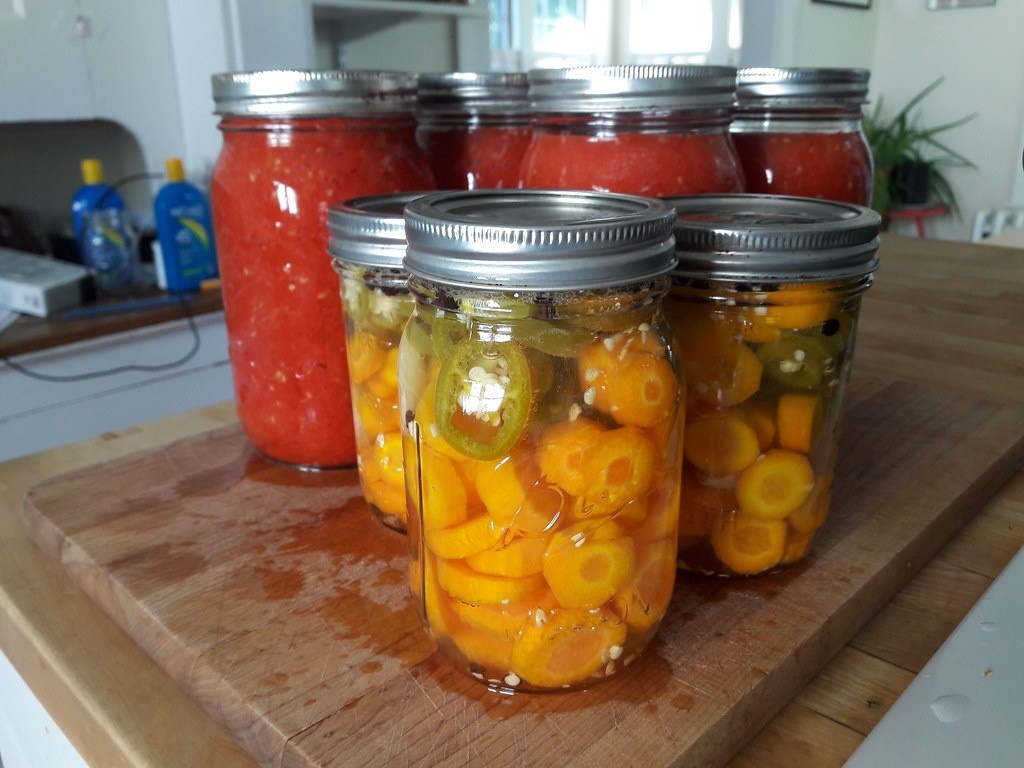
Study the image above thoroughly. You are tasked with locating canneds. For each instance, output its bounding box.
[211,63,881,693]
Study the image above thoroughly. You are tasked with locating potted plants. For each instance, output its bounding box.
[862,78,980,233]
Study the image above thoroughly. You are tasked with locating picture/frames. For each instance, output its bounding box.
[812,0,873,9]
[926,1,997,10]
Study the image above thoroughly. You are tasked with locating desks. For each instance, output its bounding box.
[1,232,1022,767]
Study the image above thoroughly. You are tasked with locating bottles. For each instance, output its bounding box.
[70,156,137,293]
[152,158,220,293]
